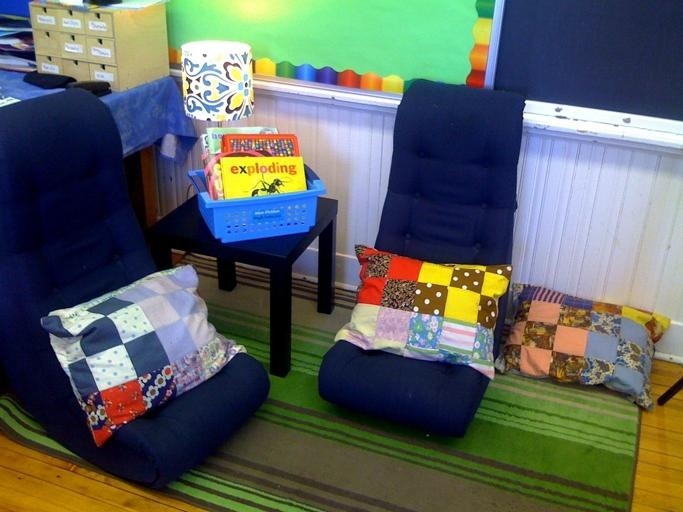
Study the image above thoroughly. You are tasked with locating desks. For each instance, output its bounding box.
[0,55,197,225]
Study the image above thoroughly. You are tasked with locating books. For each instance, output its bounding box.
[201,124,308,199]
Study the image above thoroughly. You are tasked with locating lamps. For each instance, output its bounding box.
[180,40,255,129]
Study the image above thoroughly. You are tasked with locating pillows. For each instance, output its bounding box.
[494,285,671,411]
[335,243,511,381]
[39,264,247,449]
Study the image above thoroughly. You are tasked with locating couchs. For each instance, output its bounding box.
[0,88,270,486]
[318,79,527,441]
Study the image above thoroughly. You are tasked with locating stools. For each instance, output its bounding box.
[147,188,339,378]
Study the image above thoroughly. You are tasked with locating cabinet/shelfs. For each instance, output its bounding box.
[27,0,170,94]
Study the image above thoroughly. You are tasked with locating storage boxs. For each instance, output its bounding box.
[186,162,327,243]
